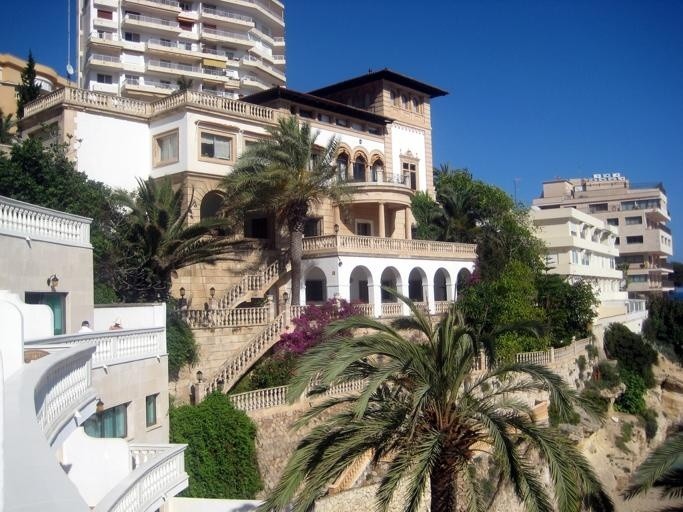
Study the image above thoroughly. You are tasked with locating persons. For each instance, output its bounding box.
[78,320,124,333]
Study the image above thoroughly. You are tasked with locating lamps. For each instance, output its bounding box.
[283,292,288,305]
[96,399,105,414]
[197,371,202,383]
[47,274,59,287]
[334,224,339,235]
[179,287,186,300]
[210,287,215,299]
